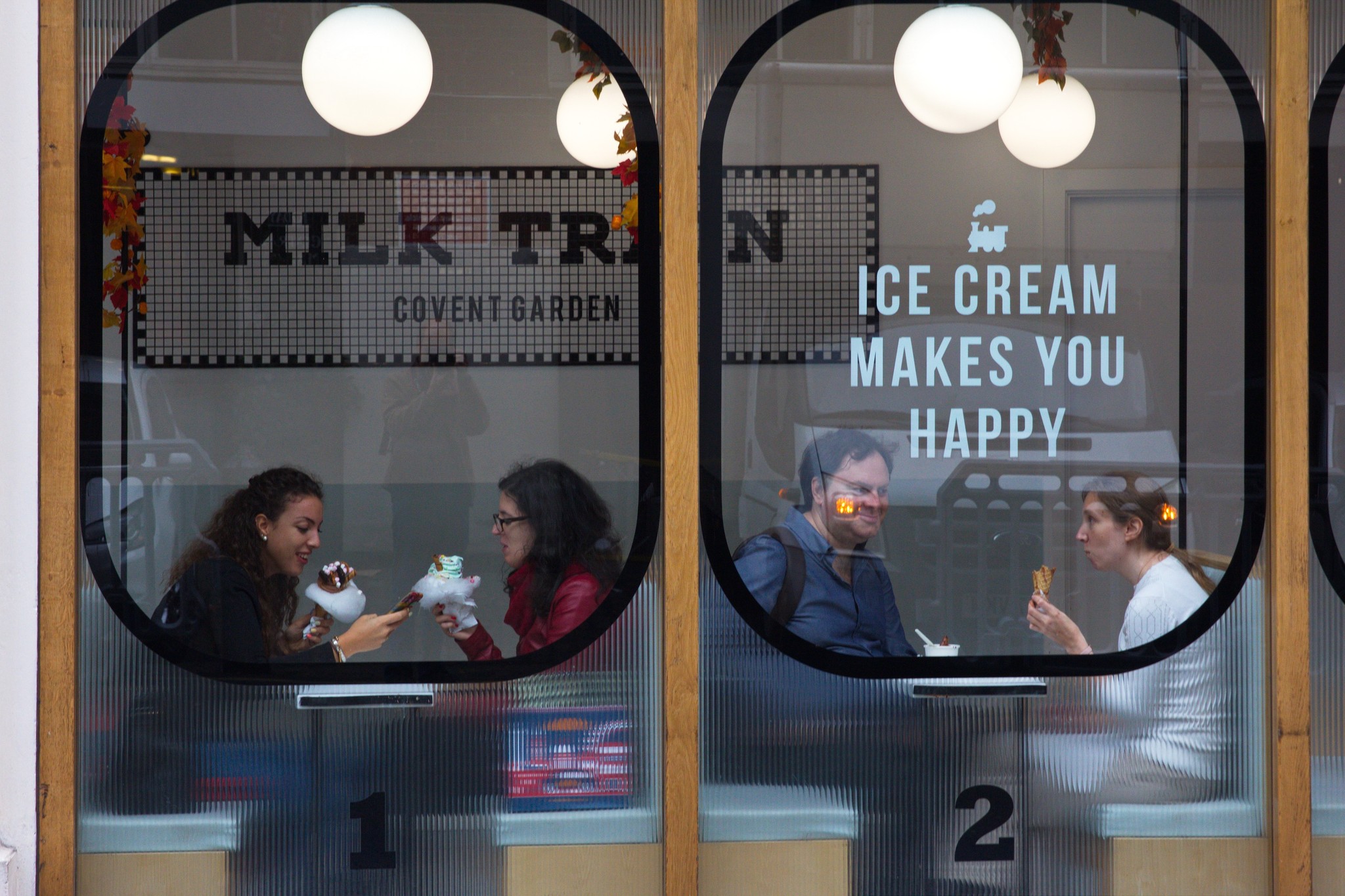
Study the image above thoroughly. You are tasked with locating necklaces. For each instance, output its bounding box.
[1128,550,1160,603]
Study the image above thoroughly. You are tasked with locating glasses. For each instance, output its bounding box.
[492,512,531,532]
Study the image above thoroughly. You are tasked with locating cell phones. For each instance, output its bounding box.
[385,591,423,615]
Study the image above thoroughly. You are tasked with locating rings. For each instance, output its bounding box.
[386,636,389,641]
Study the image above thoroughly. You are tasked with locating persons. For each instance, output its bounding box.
[356,461,630,896]
[939,470,1232,896]
[87,466,412,896]
[698,429,985,896]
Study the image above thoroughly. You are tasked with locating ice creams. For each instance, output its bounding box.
[1032,564,1057,613]
[412,554,481,633]
[303,561,366,640]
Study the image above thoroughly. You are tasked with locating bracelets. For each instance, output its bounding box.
[331,638,347,663]
[1080,647,1092,654]
[334,635,342,663]
